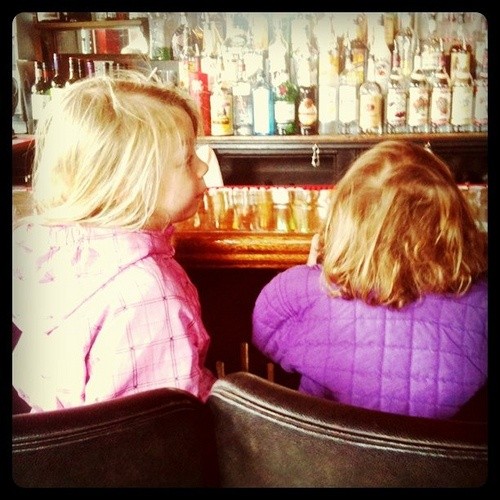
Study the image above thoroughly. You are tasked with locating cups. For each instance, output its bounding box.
[202,184,331,238]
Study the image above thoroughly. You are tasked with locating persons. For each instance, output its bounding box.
[13,79,216,411]
[252,141,488,421]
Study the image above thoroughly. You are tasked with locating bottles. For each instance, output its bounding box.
[30,11,487,135]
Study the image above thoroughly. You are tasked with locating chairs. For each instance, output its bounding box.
[12,388,218,488]
[207,370,488,488]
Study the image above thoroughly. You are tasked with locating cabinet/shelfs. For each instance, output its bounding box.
[32,12,150,91]
